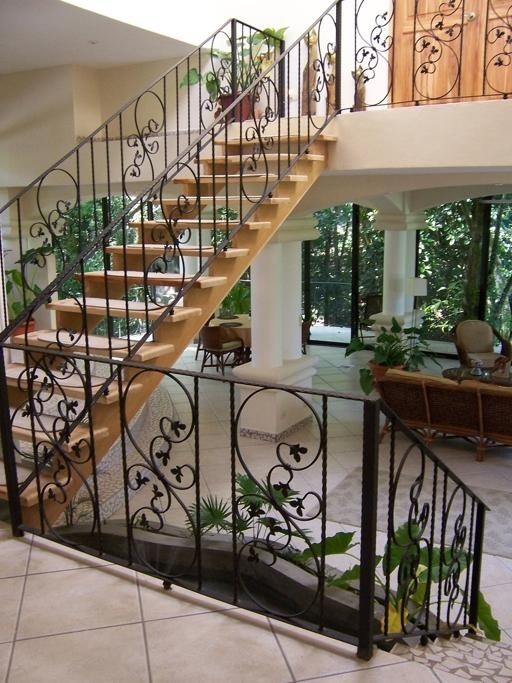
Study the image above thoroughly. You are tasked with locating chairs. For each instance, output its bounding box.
[195,311,313,382]
[452,319,512,367]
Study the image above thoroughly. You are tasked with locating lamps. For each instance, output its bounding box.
[405,276,428,355]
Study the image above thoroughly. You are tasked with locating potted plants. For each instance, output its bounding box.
[343,315,444,398]
[2,234,56,337]
[179,24,289,123]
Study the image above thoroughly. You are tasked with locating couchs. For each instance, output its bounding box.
[372,367,512,463]
